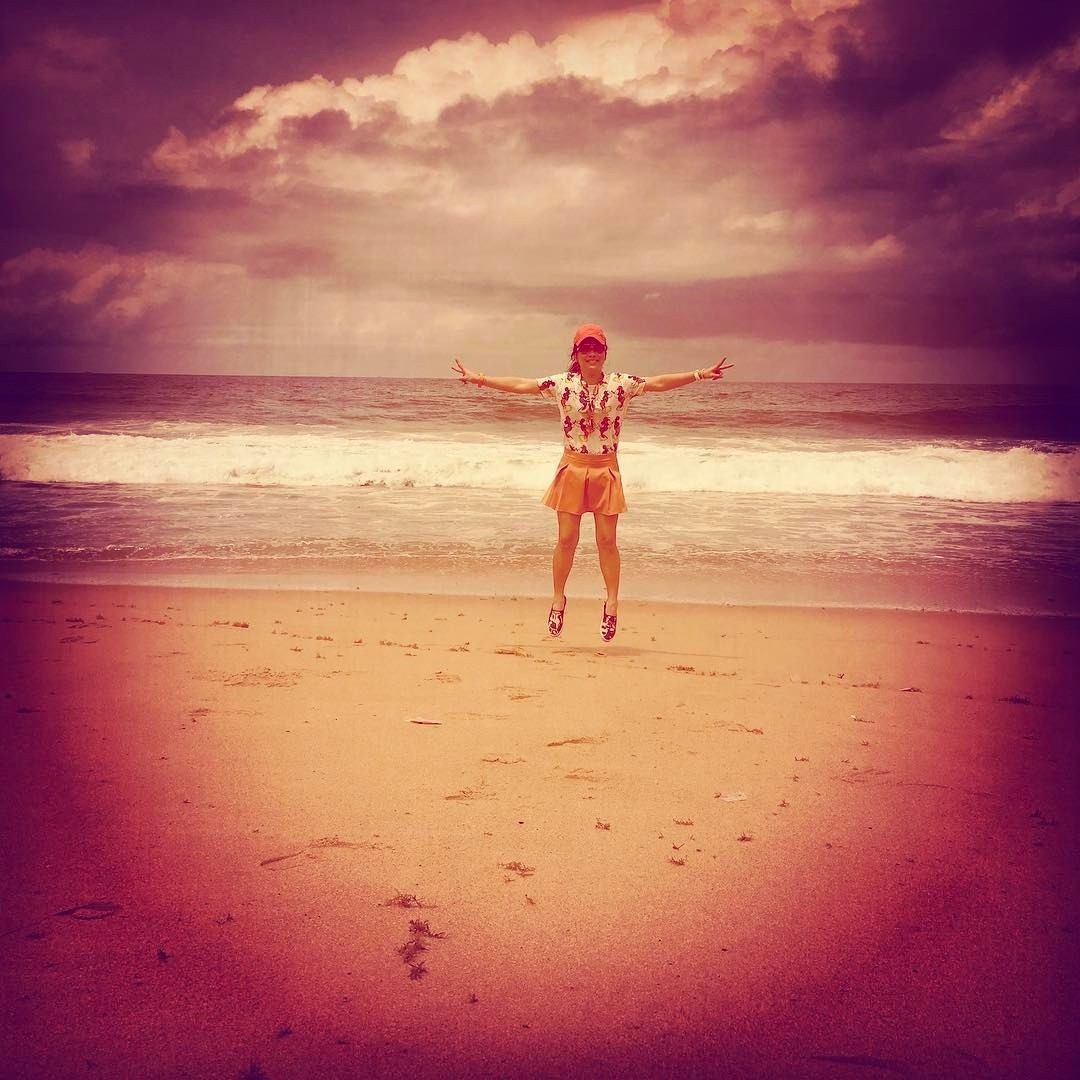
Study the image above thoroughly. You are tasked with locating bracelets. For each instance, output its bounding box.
[693,367,704,383]
[477,372,484,388]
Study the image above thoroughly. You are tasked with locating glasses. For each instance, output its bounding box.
[579,344,605,353]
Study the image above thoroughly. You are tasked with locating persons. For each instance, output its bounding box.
[451,324,736,643]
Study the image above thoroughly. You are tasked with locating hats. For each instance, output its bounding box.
[575,324,606,345]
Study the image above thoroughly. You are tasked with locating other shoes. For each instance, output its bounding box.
[601,600,618,642]
[547,596,567,636]
